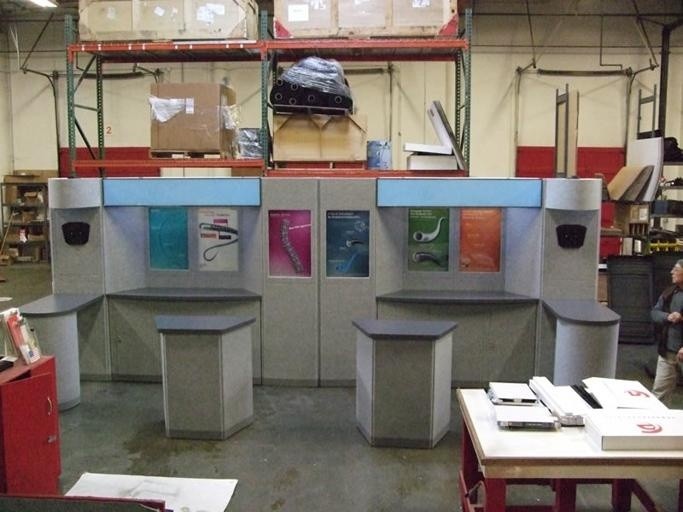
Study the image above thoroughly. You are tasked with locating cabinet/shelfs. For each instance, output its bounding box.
[0,354,62,498]
[593,138,681,347]
[0,170,60,265]
[62,2,472,174]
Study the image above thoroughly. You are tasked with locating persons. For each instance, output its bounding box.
[649,259,683,402]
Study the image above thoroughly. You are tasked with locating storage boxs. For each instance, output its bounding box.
[147,79,236,161]
[75,1,261,41]
[272,1,462,40]
[269,105,369,163]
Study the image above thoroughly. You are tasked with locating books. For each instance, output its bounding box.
[606,164,654,203]
[0,306,42,365]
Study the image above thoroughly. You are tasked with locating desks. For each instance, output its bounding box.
[153,313,260,441]
[378,288,539,388]
[20,291,109,411]
[110,286,263,387]
[455,382,682,511]
[347,315,459,447]
[541,298,622,384]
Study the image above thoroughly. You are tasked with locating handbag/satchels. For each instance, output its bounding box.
[270,56,353,115]
